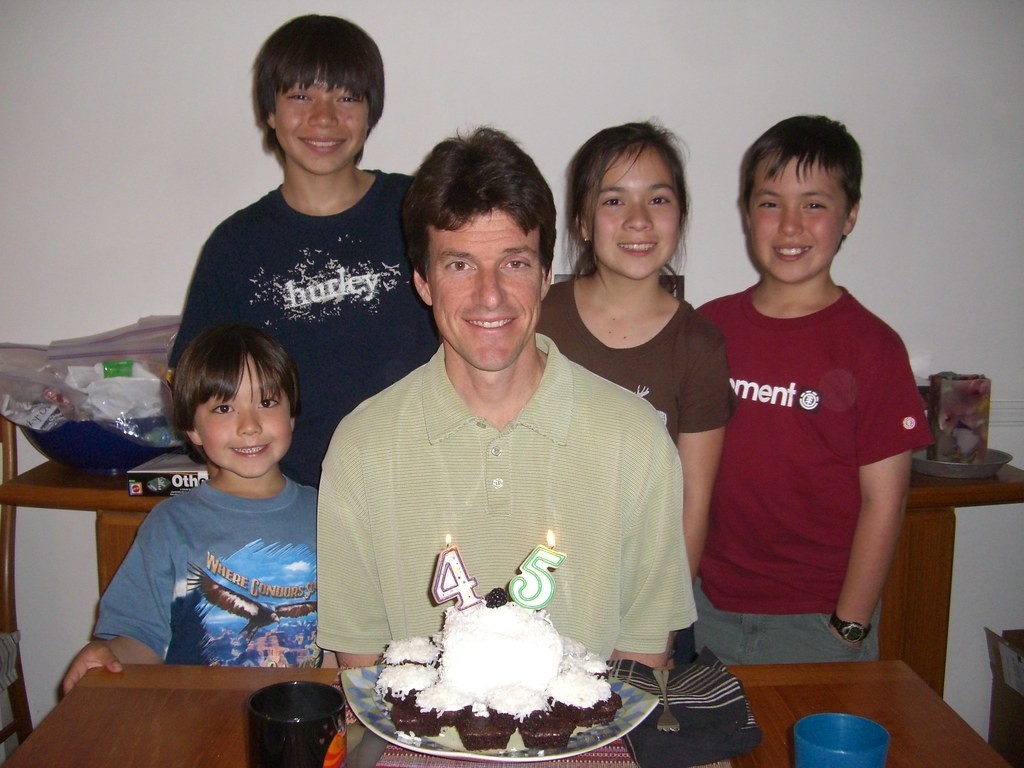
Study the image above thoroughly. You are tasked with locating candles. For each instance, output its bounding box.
[508,529,568,610]
[432,534,484,611]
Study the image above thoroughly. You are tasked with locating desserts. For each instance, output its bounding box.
[374,602,622,753]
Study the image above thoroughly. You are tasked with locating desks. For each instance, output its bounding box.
[0,663,1016,768]
[1,460,1023,698]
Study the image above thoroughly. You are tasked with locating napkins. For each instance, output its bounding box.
[606,646,764,768]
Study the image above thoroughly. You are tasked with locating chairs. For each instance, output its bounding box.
[0,413,33,744]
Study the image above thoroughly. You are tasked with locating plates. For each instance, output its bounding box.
[912,448,1015,479]
[340,663,659,763]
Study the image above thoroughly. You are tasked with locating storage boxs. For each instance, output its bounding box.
[984,625,1024,768]
[126,454,209,496]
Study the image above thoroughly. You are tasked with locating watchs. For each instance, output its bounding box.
[830,610,873,642]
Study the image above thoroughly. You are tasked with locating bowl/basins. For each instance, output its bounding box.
[19,414,167,475]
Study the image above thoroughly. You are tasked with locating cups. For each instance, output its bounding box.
[246,680,347,768]
[793,713,891,768]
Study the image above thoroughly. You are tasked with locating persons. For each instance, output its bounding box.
[64,323,340,694]
[693,118,910,664]
[169,16,442,492]
[317,130,673,666]
[540,125,724,667]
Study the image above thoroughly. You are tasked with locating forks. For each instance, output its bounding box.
[652,669,680,733]
